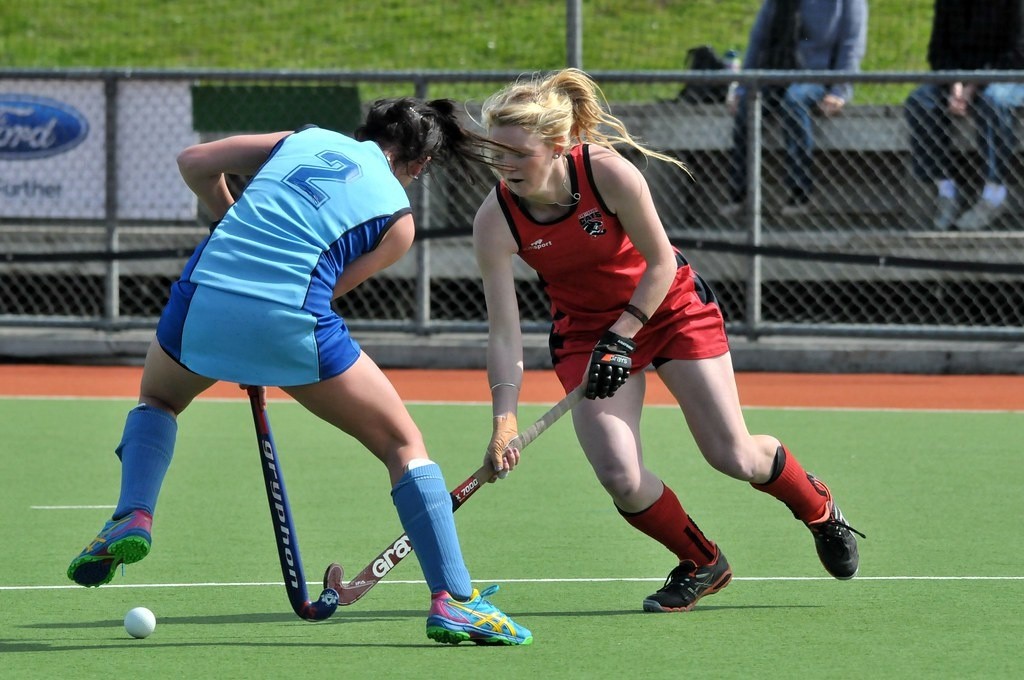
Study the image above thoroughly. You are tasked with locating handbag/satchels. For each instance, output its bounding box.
[679,47,728,104]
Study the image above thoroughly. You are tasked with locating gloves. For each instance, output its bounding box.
[581,331,636,402]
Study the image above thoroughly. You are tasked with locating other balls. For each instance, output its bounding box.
[123,607,156,639]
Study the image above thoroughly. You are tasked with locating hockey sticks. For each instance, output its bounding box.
[321,384,586,607]
[244,384,339,623]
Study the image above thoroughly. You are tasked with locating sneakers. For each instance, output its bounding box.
[643,542,732,614]
[801,471,867,581]
[66,509,152,588]
[426,584,533,648]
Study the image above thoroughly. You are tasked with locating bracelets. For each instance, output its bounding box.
[491,382,520,391]
[625,303,649,326]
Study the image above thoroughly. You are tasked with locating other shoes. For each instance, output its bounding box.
[722,193,749,217]
[958,196,1009,232]
[934,181,963,230]
[782,189,810,217]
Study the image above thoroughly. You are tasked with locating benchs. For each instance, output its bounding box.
[363,103,1023,229]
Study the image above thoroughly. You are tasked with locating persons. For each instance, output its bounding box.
[67,97,533,648]
[903,0,1024,233]
[717,1,868,223]
[474,68,865,613]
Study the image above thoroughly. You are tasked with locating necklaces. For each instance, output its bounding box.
[527,157,581,206]
